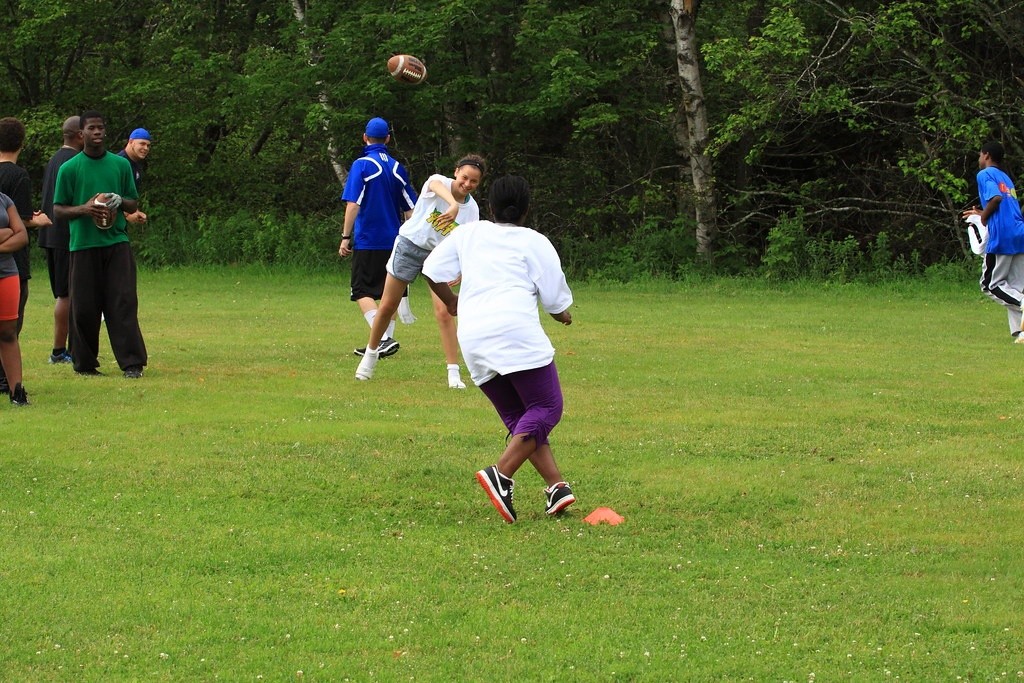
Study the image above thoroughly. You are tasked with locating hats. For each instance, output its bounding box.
[130,128,151,140]
[366,117,389,138]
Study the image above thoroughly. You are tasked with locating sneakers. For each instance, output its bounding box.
[544,482,575,515]
[123,366,144,378]
[48,350,73,364]
[354,337,400,359]
[477,464,517,524]
[78,369,105,377]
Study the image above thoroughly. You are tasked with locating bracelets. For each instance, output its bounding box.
[125,213,130,217]
[342,234,351,239]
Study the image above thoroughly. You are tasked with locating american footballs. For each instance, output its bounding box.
[386,55,429,86]
[93,193,118,231]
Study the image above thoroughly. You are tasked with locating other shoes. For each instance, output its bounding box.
[1015,332,1024,345]
[10,383,30,406]
[0,376,12,395]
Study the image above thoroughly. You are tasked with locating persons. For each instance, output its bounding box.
[356,153,487,388]
[0,117,53,404]
[963,141,1024,344]
[339,117,417,360]
[39,115,84,365]
[422,170,575,523]
[53,110,147,378]
[115,128,150,224]
